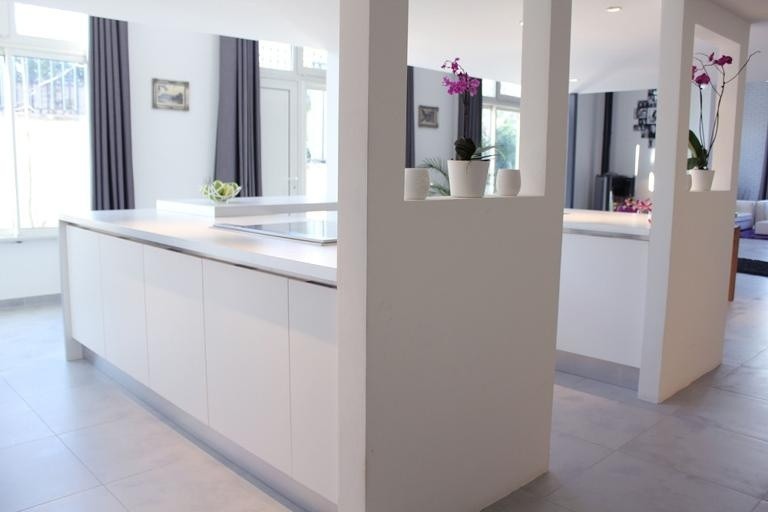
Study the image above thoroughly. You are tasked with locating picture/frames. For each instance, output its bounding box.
[418,105,438,127]
[152,77,190,110]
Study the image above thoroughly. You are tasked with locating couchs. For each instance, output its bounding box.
[734,199,768,236]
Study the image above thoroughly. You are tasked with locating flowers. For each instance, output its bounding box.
[688,49,763,167]
[616,196,654,215]
[439,57,485,160]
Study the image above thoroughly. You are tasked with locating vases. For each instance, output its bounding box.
[404,161,521,200]
[690,169,715,192]
